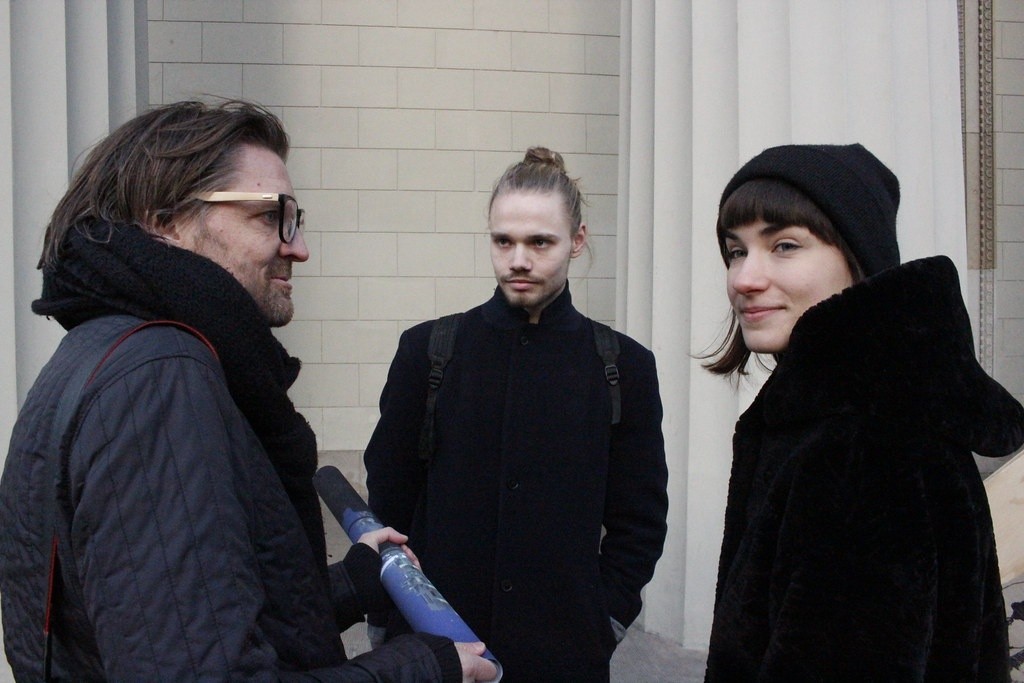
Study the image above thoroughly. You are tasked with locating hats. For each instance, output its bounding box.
[718,143,900,277]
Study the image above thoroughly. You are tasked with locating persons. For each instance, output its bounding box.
[365,146,670,682]
[687,140,1024,682]
[2,95,506,682]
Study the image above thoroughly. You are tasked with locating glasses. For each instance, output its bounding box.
[192,192,305,244]
